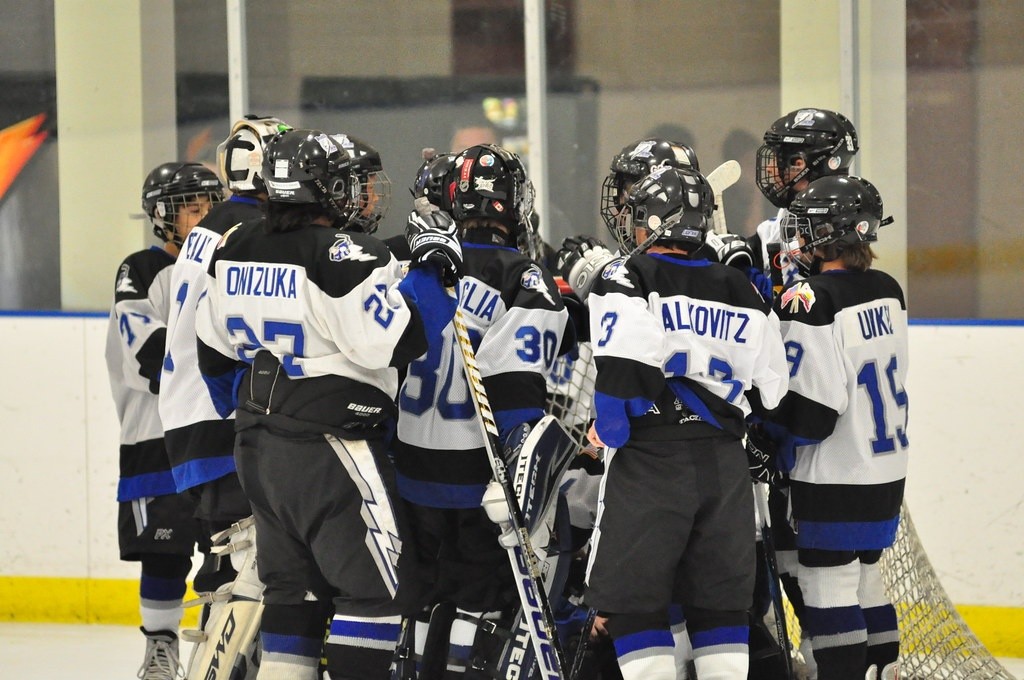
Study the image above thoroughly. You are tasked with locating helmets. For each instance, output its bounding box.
[756,107,860,209]
[779,174,886,277]
[295,131,394,231]
[406,147,459,207]
[617,166,718,246]
[260,128,323,211]
[599,137,701,192]
[217,114,292,194]
[449,141,536,230]
[142,161,221,251]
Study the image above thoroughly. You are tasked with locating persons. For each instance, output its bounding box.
[103,107,910,680]
[587,167,790,680]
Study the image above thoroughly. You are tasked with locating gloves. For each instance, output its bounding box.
[708,231,755,268]
[553,234,626,304]
[745,430,788,491]
[405,198,464,290]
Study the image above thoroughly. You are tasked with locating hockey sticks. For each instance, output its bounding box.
[418,191,565,680]
[703,157,796,668]
[765,241,785,300]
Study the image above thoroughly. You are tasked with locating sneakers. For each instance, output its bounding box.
[138,624,186,680]
